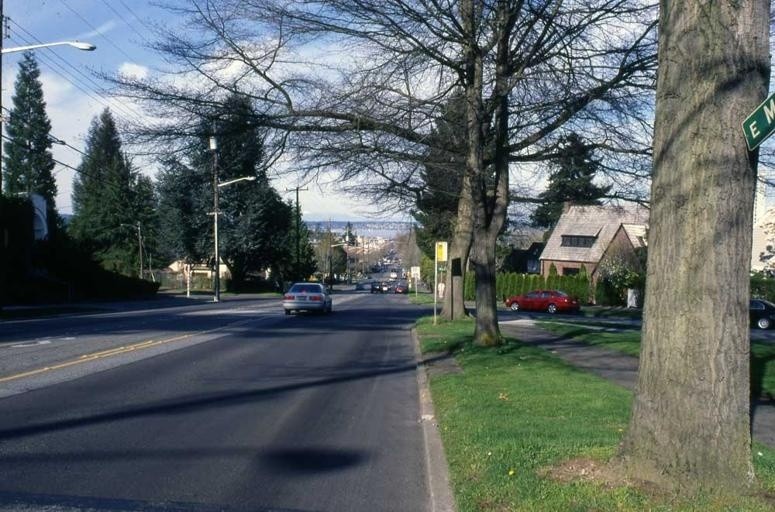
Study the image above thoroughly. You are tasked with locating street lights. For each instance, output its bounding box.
[330,244,368,291]
[1,41,96,54]
[209,135,257,303]
[120,221,144,279]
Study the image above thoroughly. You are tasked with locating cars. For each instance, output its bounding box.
[505,290,581,314]
[750,298,775,330]
[371,271,409,294]
[282,283,332,315]
